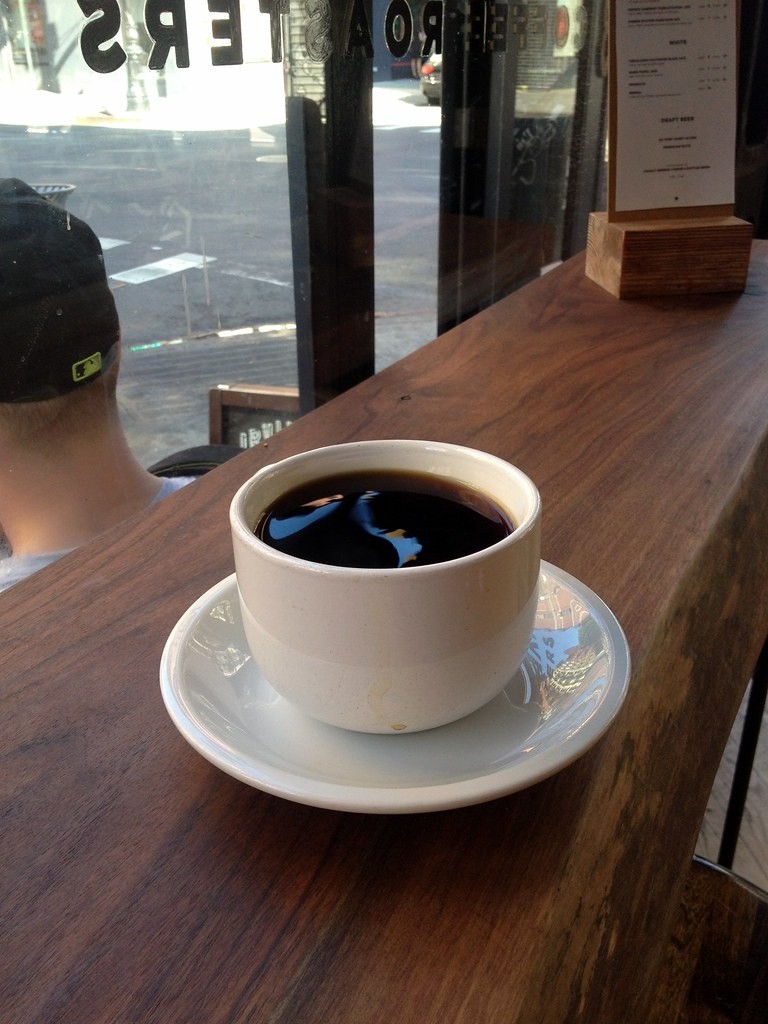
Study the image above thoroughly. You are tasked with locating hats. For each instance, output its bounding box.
[0,177,121,403]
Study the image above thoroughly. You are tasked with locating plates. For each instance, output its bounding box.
[158,559,633,815]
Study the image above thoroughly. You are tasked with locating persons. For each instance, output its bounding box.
[0,174,201,630]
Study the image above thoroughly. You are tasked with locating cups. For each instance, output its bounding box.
[229,438,542,737]
